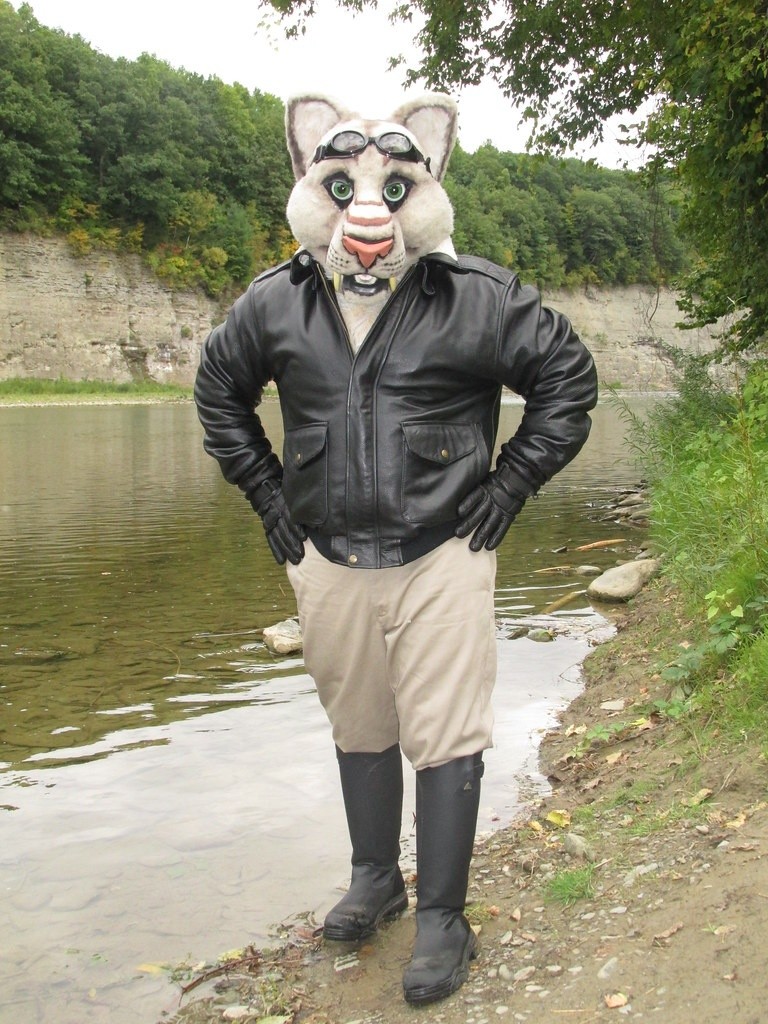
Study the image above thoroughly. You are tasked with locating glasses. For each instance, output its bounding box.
[313,130,425,172]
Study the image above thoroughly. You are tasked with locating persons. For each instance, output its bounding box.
[194,246,597,1003]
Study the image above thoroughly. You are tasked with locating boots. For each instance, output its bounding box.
[403,751,485,997]
[324,742,407,944]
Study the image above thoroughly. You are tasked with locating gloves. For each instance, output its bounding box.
[455,463,536,552]
[245,477,307,567]
